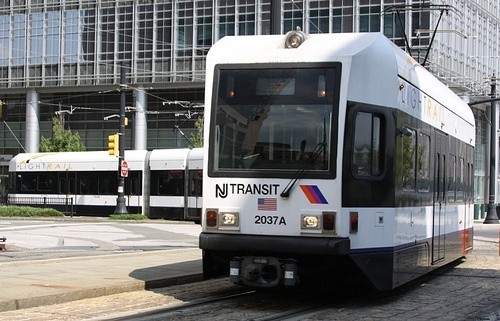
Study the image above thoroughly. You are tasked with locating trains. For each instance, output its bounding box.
[7,31,476,300]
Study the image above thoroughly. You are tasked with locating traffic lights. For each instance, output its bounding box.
[108,133,119,158]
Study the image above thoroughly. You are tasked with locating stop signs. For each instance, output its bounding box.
[120,160,128,177]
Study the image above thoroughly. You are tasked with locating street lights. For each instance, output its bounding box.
[483,72,500,225]
